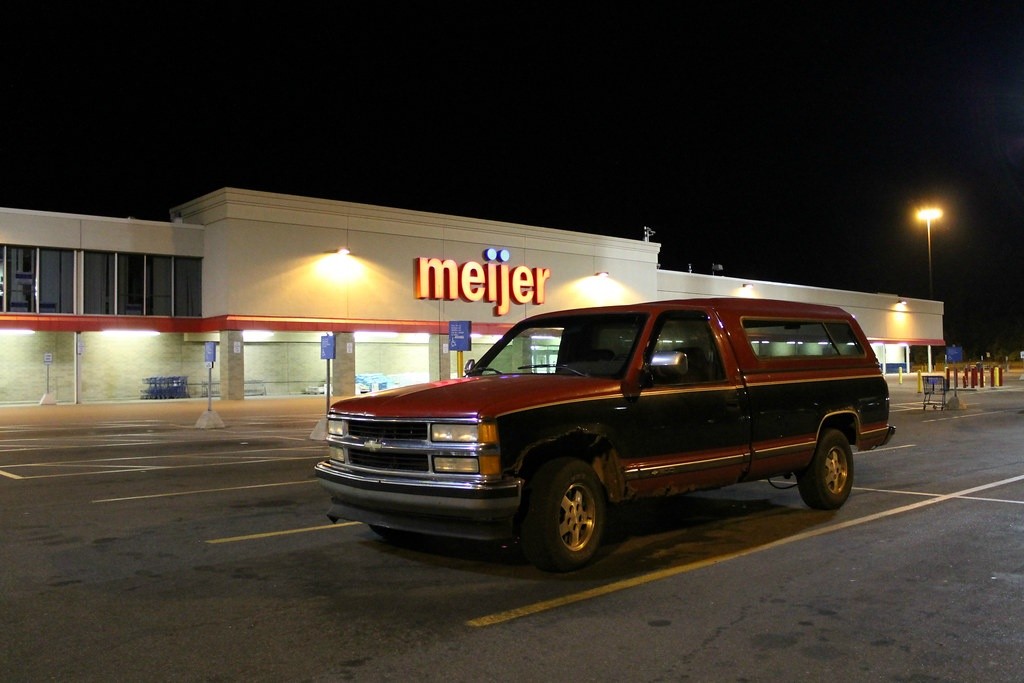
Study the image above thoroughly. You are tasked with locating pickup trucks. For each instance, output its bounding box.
[314,298,897,573]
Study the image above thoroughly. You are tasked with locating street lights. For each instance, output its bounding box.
[915,207,943,300]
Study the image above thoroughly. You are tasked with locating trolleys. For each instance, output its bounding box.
[139,376,189,400]
[922,376,947,411]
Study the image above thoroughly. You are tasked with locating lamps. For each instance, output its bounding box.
[711,264,723,276]
[897,300,907,305]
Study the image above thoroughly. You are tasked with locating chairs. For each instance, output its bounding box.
[671,345,711,384]
